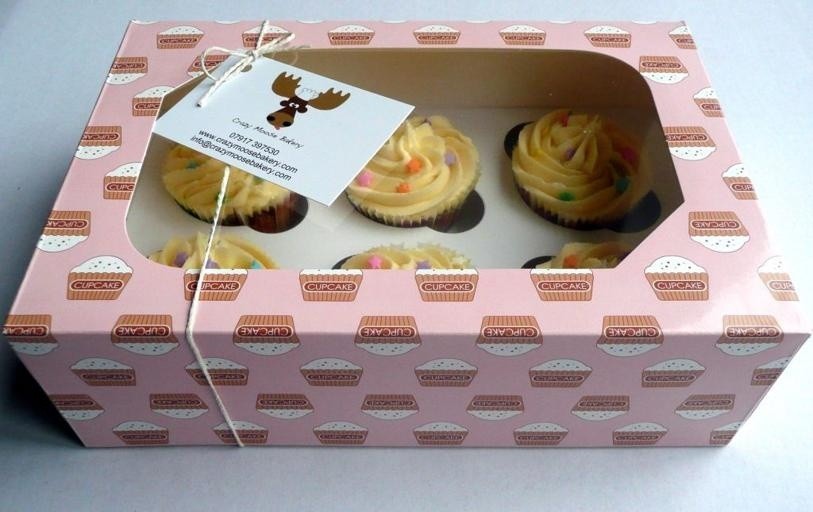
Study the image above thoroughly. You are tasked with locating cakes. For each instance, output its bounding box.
[339,241,470,271]
[339,112,481,232]
[533,237,623,269]
[512,106,654,229]
[142,234,270,272]
[157,143,298,231]
